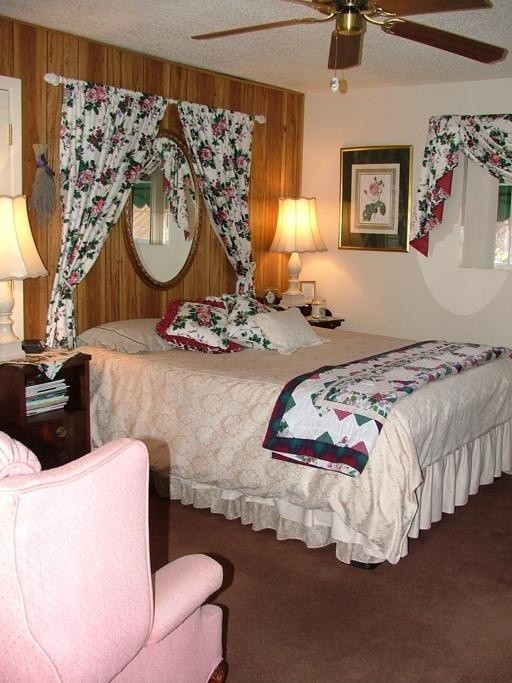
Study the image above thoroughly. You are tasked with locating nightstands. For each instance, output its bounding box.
[1,351,92,473]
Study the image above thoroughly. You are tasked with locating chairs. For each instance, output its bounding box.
[1,433,229,683]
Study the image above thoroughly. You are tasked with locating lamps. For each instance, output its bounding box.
[269,196,328,307]
[0,193,50,365]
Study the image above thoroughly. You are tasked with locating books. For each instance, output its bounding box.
[23,377,73,418]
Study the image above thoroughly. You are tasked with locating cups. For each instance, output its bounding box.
[310,297,328,319]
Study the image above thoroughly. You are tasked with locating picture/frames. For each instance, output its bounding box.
[338,145,414,253]
[300,281,316,305]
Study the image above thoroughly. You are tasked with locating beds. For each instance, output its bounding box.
[73,319,512,567]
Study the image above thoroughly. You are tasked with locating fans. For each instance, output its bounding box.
[192,1,506,70]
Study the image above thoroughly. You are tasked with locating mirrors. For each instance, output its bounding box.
[123,130,204,290]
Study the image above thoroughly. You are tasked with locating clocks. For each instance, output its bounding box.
[266,291,276,303]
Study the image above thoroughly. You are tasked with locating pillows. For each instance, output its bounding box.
[76,293,332,357]
